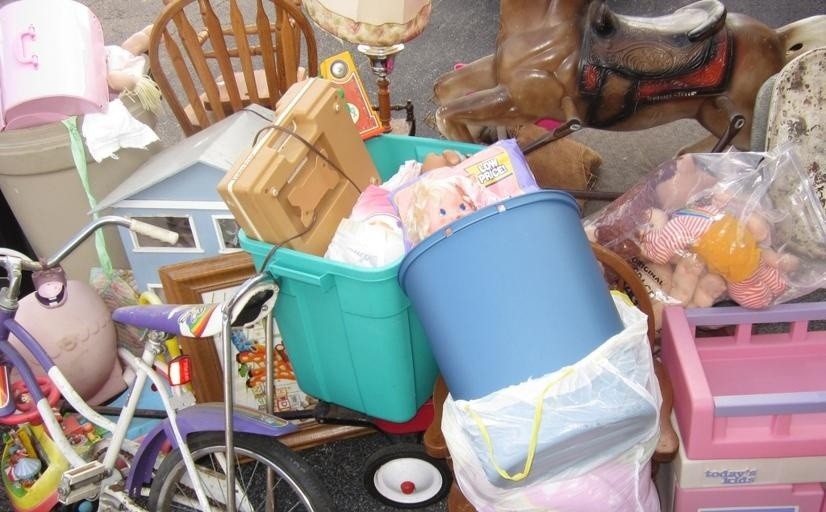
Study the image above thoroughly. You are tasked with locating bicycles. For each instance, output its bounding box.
[0,213,338,511]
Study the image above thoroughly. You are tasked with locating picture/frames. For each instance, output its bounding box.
[158,255,378,465]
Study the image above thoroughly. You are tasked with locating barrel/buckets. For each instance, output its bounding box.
[0,92,160,281]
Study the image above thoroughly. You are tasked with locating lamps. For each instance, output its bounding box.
[301,0,434,137]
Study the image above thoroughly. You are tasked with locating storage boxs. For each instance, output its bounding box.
[236,133,487,425]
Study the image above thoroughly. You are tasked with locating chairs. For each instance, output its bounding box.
[149,0,318,139]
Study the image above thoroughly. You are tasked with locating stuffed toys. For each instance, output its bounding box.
[580,154,803,343]
[399,174,502,249]
